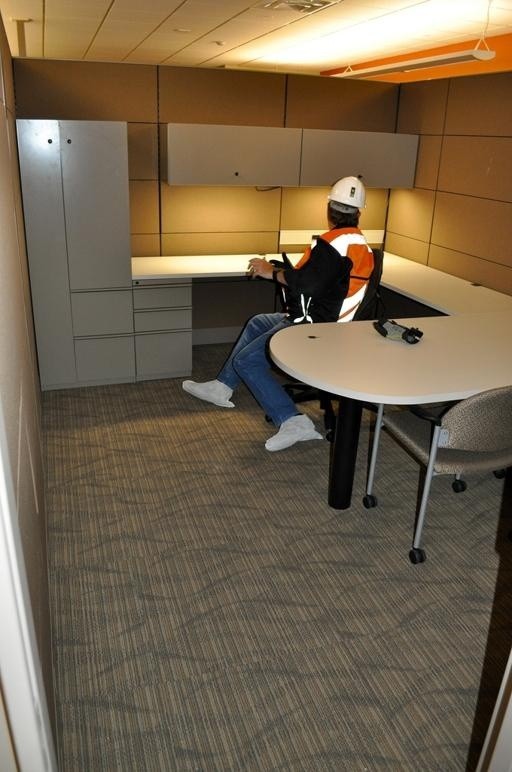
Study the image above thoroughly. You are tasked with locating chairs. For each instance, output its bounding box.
[362,385,512,563]
[269,249,383,443]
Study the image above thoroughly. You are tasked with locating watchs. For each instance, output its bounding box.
[272,266,281,280]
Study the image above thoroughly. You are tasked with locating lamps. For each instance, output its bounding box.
[331,1,496,80]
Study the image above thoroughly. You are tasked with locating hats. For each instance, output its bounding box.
[327,176,366,209]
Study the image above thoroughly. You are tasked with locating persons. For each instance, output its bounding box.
[180,175,376,453]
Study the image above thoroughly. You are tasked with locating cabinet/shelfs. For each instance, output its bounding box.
[70,288,136,388]
[300,129,420,189]
[15,118,78,393]
[133,279,192,383]
[59,119,132,293]
[167,123,304,188]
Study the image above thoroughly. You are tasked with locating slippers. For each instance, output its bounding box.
[264,413,316,451]
[181,378,236,408]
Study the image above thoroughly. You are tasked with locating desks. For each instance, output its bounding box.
[269,297,512,510]
[131,251,512,316]
